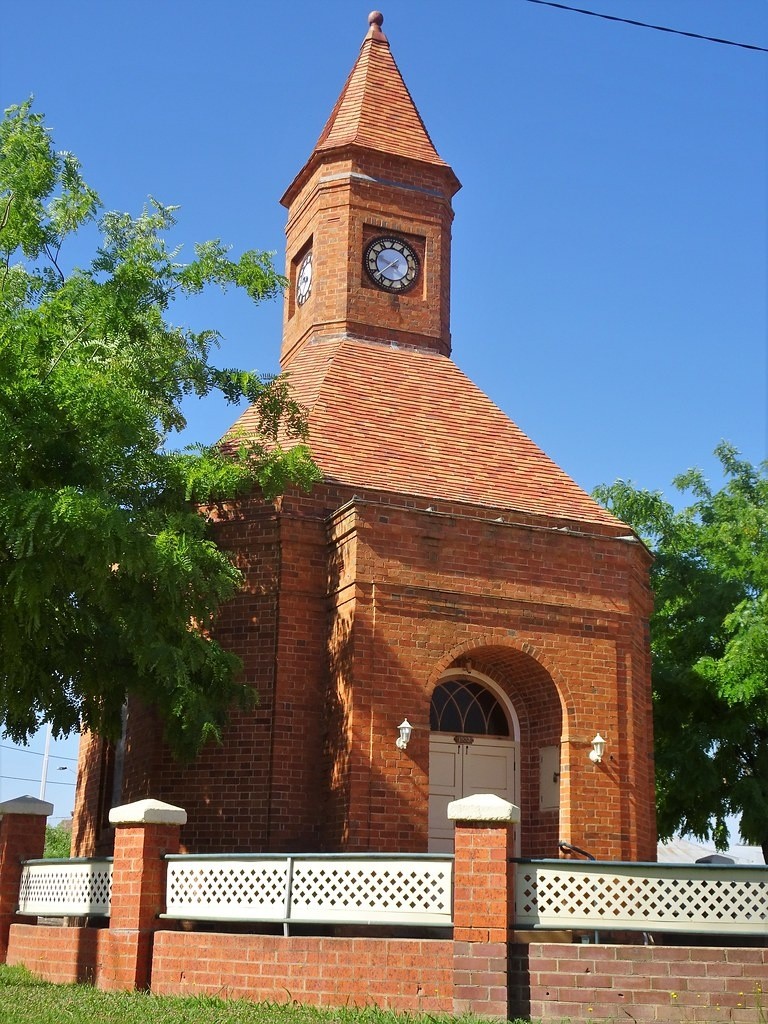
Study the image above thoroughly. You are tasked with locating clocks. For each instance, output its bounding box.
[363,236,421,294]
[296,252,313,307]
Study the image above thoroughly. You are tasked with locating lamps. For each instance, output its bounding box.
[589,732,606,764]
[396,718,413,750]
